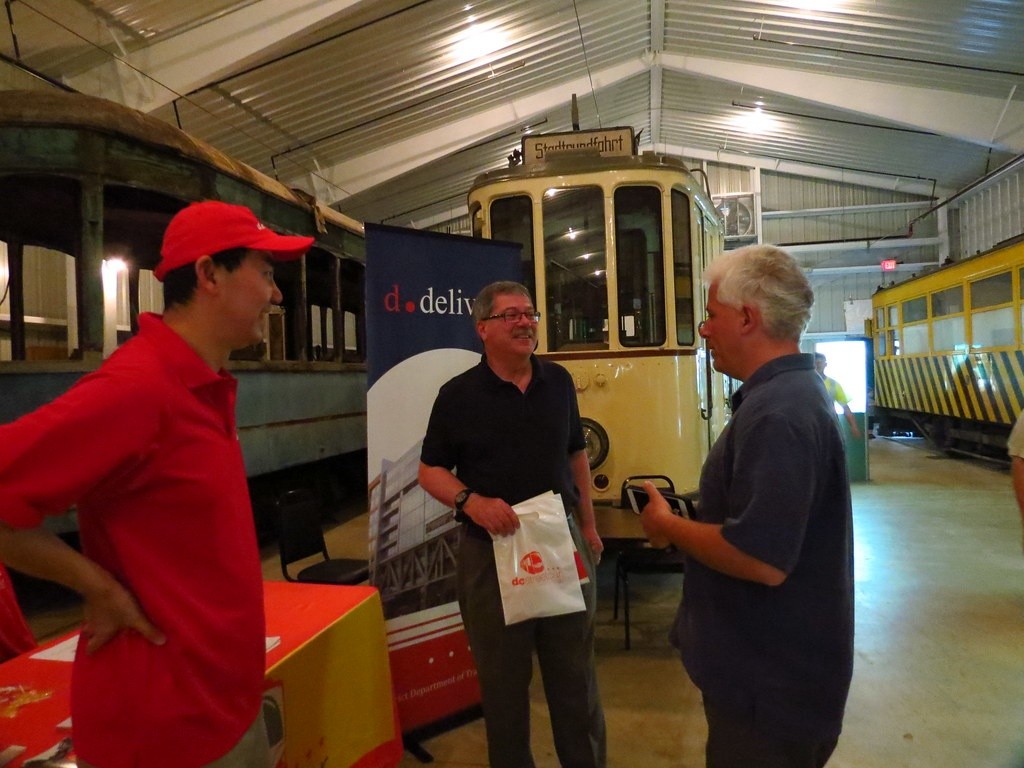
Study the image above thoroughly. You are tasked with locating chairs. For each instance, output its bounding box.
[588,505,656,650]
[274,489,370,587]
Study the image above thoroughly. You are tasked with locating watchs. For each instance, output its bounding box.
[456,488,479,512]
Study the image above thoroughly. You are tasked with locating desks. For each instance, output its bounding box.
[1,580,404,768]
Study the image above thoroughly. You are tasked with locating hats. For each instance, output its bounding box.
[157,202,314,278]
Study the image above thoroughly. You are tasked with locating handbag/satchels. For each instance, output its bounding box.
[485,491,589,628]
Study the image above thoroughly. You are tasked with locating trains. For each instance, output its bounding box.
[865,229,1023,465]
[0,83,382,541]
[468,95,730,546]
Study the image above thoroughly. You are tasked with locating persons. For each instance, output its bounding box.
[813,353,861,438]
[638,245,854,768]
[0,201,316,768]
[1007,409,1024,521]
[417,283,609,768]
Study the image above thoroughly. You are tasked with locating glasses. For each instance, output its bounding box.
[482,310,543,327]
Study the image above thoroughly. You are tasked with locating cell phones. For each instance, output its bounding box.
[626,486,695,520]
